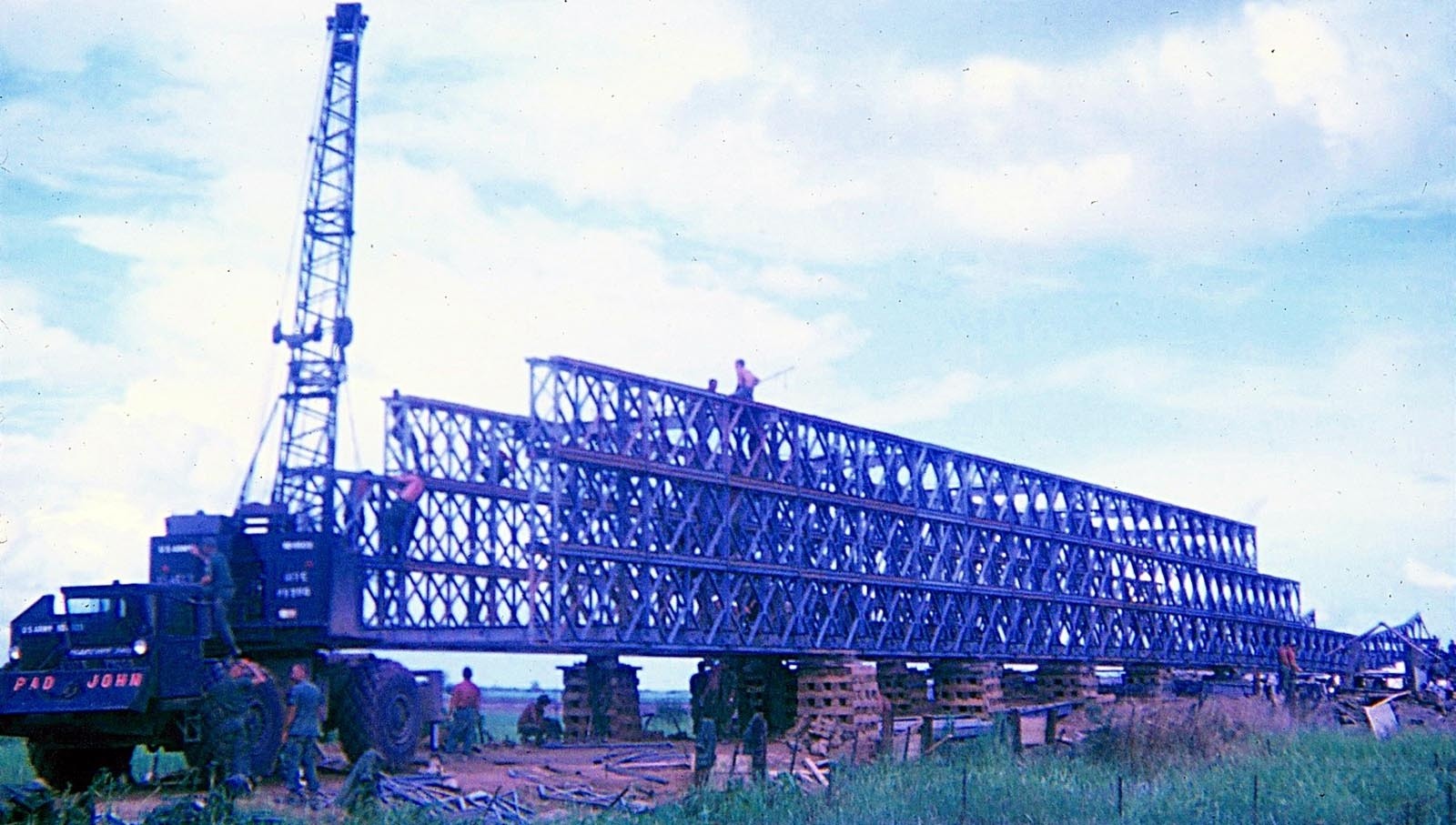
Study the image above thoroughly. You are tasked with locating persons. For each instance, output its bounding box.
[1449,640,1456,657]
[192,537,240,656]
[1278,641,1302,687]
[380,468,424,559]
[518,695,551,745]
[283,665,321,795]
[690,658,738,741]
[208,659,265,780]
[345,470,376,542]
[708,379,717,392]
[729,360,759,401]
[445,667,482,760]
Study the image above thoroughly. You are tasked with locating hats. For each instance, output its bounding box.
[535,695,551,705]
[220,654,243,671]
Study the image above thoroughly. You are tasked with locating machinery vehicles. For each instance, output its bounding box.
[0,1,428,787]
